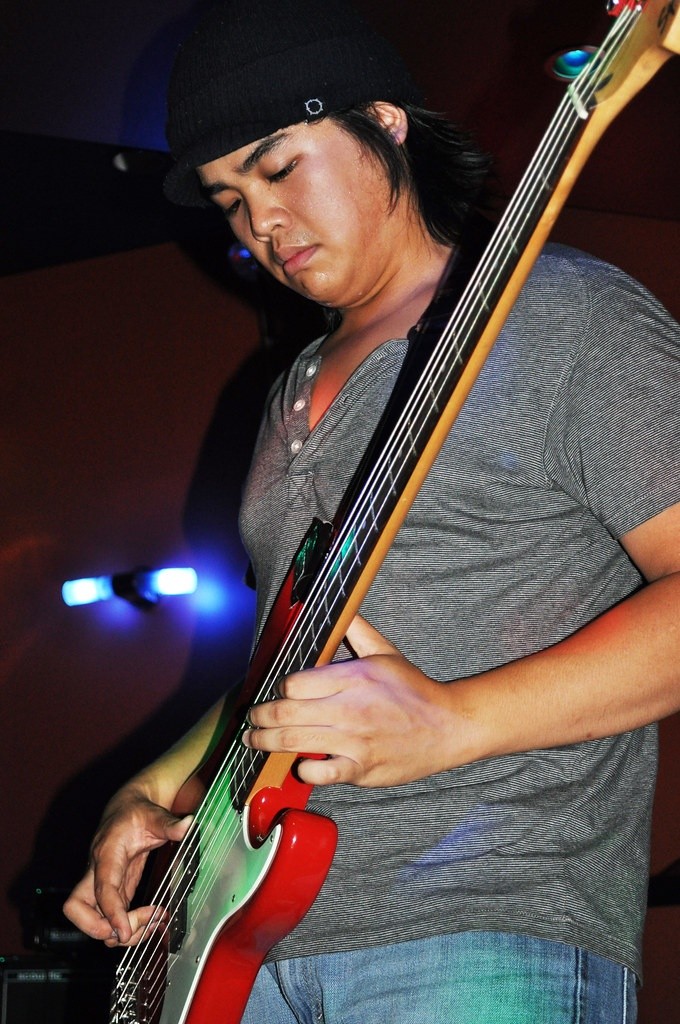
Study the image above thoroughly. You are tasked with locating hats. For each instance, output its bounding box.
[162,0,423,207]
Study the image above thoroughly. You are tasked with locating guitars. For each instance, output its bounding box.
[102,0,673,1022]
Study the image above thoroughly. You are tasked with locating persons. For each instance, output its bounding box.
[63,0,680,1024]
